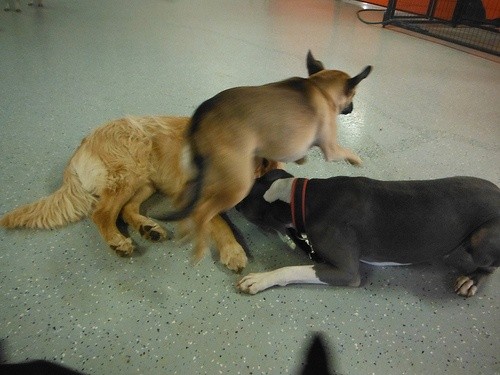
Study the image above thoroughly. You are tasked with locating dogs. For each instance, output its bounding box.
[0,112,284,274]
[233,168,500,298]
[148,49,374,269]
